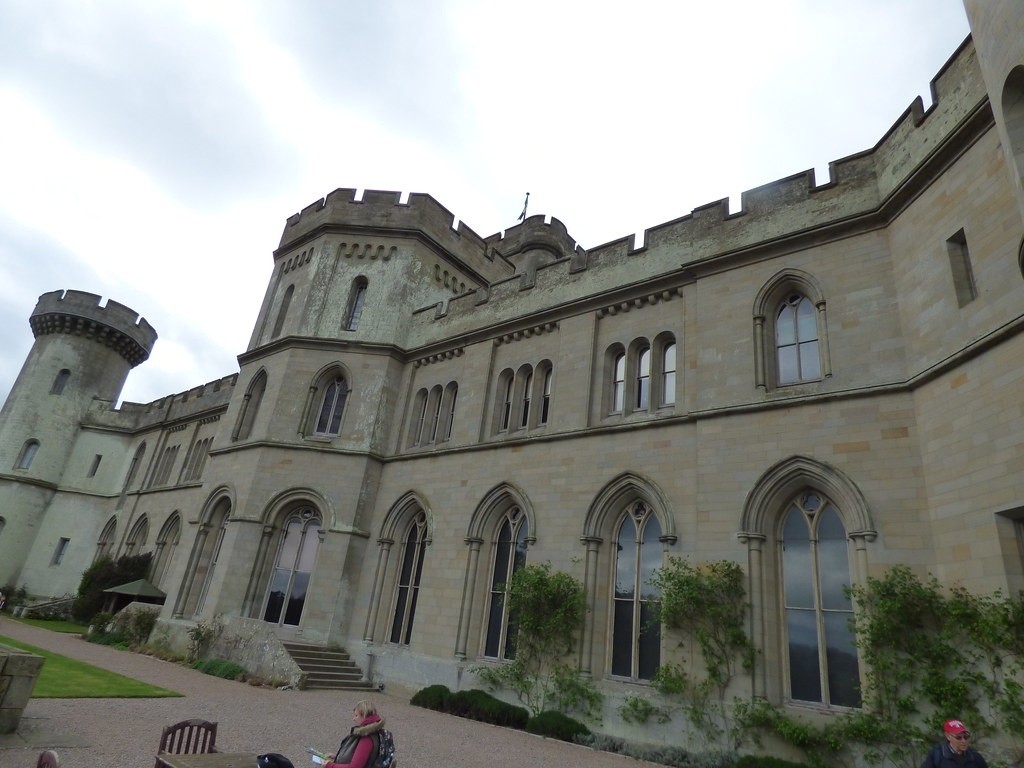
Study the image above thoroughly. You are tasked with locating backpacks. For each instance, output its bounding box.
[373,729,395,768]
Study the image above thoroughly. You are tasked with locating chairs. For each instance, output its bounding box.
[156,718,222,768]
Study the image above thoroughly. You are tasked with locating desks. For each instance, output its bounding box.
[155,752,258,768]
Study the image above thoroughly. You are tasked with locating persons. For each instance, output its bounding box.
[0,591,5,609]
[37,750,60,768]
[322,700,385,768]
[920,719,989,768]
